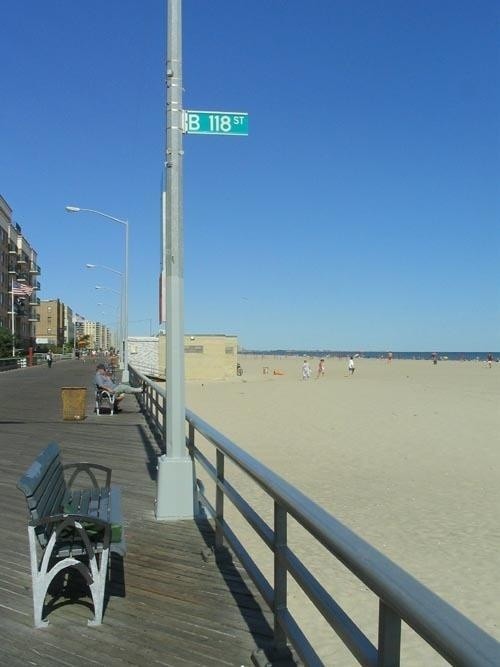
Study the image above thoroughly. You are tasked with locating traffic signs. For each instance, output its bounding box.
[187,110,249,137]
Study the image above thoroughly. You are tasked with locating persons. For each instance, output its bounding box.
[96,363,145,415]
[317,359,325,378]
[301,360,311,381]
[81,347,120,365]
[348,357,355,376]
[431,352,437,365]
[388,351,393,366]
[46,348,54,368]
[488,353,494,367]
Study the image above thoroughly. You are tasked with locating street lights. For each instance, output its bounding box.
[65,206,129,371]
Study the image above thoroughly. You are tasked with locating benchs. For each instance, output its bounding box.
[96,383,124,417]
[16,439,126,628]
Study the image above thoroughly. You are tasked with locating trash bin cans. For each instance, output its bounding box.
[61,387,87,421]
